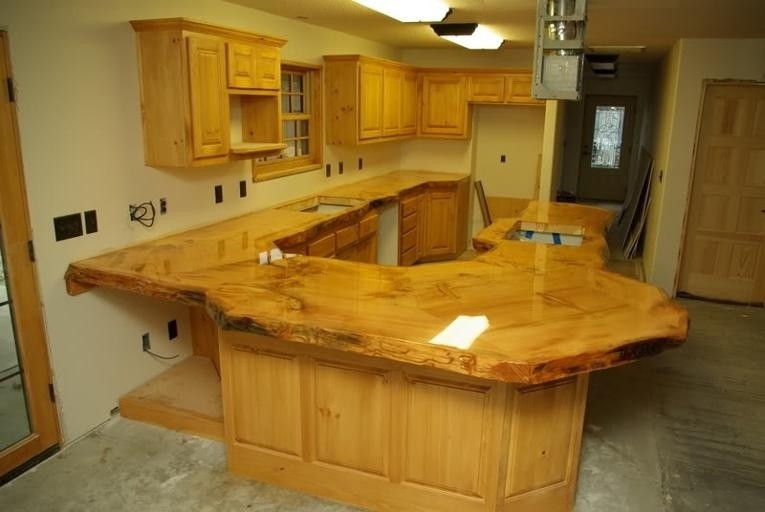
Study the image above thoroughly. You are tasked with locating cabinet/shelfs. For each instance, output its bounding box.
[129,18,289,169]
[322,53,473,147]
[219,252,391,512]
[401,183,470,266]
[308,213,379,264]
[390,249,492,511]
[492,239,595,512]
[467,69,545,106]
